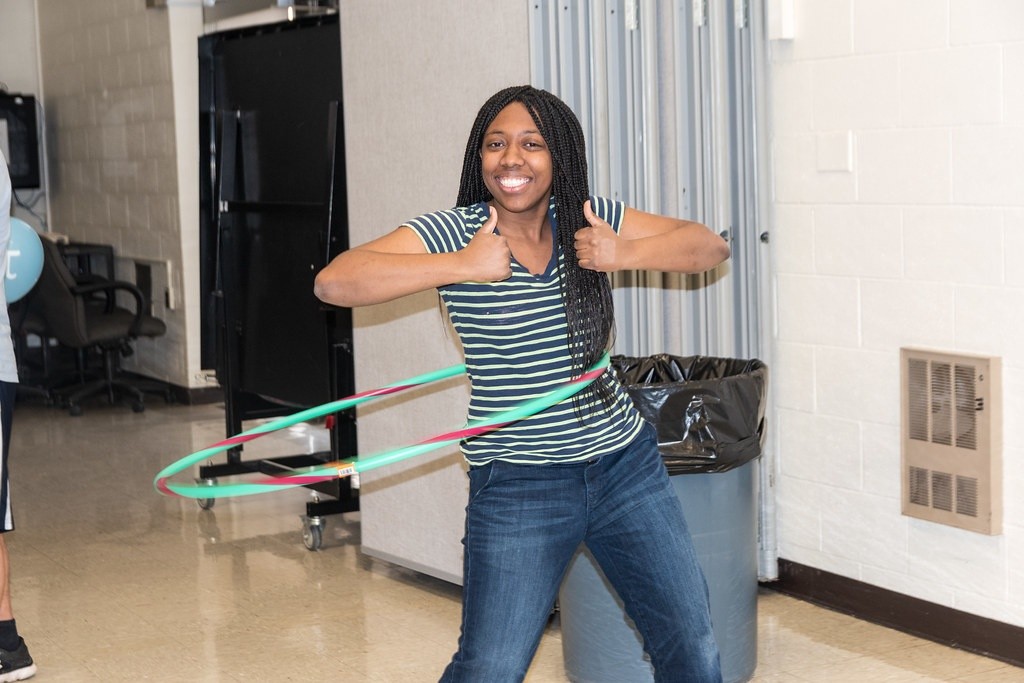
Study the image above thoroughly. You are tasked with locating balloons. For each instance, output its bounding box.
[3,215,45,303]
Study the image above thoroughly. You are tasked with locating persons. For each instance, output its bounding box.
[0,149,37,682]
[315,85,730,683]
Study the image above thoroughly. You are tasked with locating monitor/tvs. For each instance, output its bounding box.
[0,94,40,189]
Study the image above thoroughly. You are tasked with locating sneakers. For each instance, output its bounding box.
[0,636,37,683]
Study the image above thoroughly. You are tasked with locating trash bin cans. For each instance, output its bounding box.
[560,353,772,683]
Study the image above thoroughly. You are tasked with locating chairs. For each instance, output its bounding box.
[37,234,176,417]
